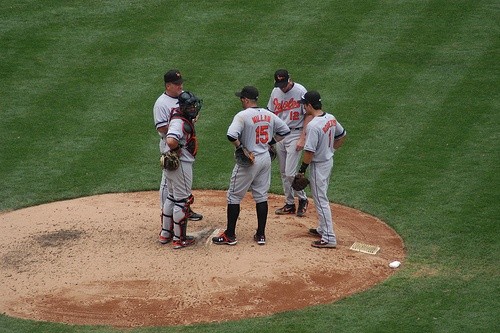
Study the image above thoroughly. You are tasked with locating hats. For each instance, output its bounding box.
[274,69,289,87]
[164,70,184,84]
[234,86,257,99]
[298,92,321,104]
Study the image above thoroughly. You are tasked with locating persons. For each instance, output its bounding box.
[213,85,292,245]
[296,90,347,249]
[266,69,308,217]
[152,70,203,249]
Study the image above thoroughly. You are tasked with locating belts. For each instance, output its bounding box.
[289,127,301,131]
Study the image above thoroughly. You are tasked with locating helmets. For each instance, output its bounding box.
[178,92,196,107]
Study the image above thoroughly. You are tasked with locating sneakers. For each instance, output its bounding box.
[275,204,296,214]
[254,233,265,244]
[188,209,202,220]
[159,231,175,244]
[311,240,336,248]
[298,199,308,216]
[172,235,194,248]
[212,230,237,245]
[309,229,318,235]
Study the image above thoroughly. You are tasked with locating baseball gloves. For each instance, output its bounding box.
[233,144,255,168]
[269,146,276,162]
[292,172,310,191]
[159,150,180,171]
